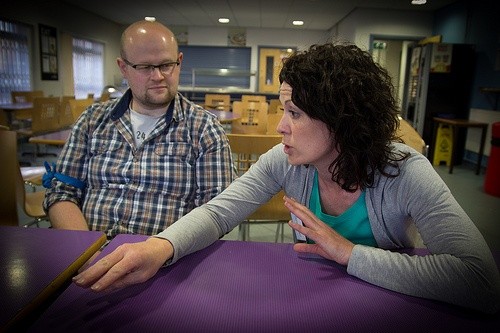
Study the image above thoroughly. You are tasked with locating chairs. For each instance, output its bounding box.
[0,90,426,242]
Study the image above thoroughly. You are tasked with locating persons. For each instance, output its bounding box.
[42,19,239,240]
[72,40,500,307]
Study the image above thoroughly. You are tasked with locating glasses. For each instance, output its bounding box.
[122,57,180,73]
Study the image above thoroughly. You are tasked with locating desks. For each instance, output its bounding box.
[0,225,500,333]
[428,116,489,176]
[207,108,239,134]
[28,130,72,166]
[0,101,32,128]
